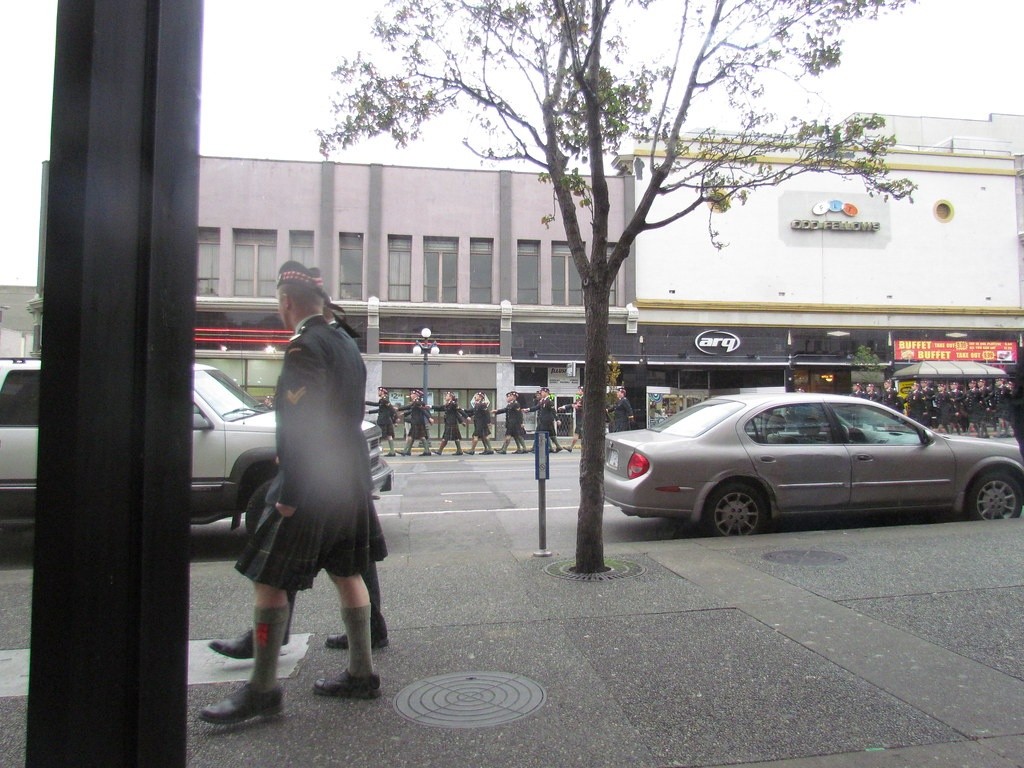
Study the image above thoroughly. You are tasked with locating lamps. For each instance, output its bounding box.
[530,348,538,359]
[677,349,690,361]
[746,351,761,361]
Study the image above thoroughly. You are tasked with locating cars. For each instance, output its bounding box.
[601,392,1024,536]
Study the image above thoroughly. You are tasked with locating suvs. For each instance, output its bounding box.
[0,356,393,525]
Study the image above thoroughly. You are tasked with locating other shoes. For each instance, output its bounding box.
[977,428,1015,438]
[384,447,562,457]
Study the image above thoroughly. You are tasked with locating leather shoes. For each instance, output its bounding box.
[325,613,389,649]
[563,446,572,452]
[198,682,283,724]
[209,629,289,659]
[313,668,382,700]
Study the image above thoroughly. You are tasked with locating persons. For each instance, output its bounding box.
[520,388,562,453]
[492,391,529,454]
[393,390,434,456]
[424,392,472,454]
[462,391,493,454]
[563,386,584,450]
[365,386,400,456]
[608,389,635,433]
[849,378,1017,438]
[198,262,388,723]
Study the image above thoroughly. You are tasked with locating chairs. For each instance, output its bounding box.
[797,417,827,443]
[766,414,797,443]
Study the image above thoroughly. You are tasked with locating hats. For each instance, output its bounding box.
[850,377,1017,391]
[378,387,388,394]
[477,391,484,399]
[446,391,454,398]
[506,391,519,398]
[276,260,324,296]
[539,388,550,393]
[578,386,583,390]
[617,388,627,396]
[410,389,424,397]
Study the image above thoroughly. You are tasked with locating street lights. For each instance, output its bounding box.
[414,327,439,407]
